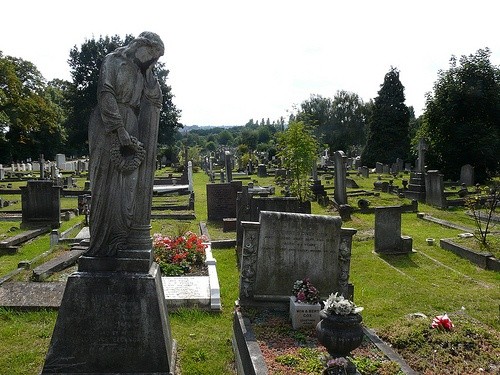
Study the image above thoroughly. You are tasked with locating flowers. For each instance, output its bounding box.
[326,355,349,375]
[323,292,364,316]
[292,278,320,305]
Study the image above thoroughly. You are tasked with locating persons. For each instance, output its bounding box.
[84,32,165,256]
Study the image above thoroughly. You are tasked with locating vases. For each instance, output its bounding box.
[315,310,364,358]
